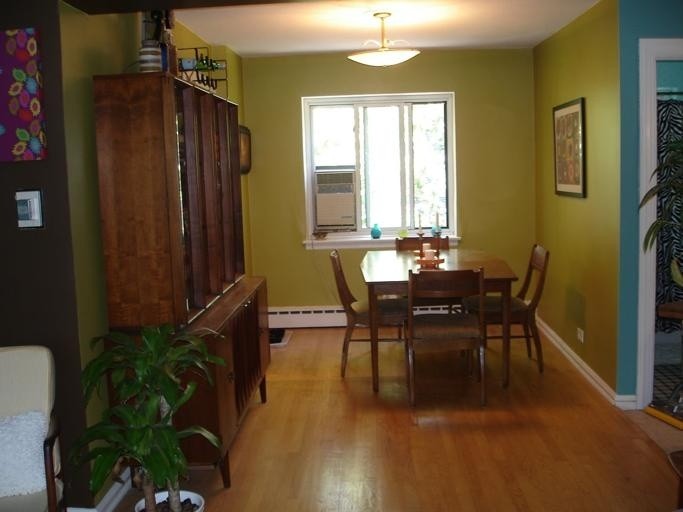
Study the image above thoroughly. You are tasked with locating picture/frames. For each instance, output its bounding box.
[553,97,586,199]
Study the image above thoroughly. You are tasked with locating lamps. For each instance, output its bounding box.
[347,11,421,67]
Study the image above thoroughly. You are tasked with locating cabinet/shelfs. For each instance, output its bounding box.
[93,74,271,489]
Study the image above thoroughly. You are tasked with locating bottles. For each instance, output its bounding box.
[371,224,382,239]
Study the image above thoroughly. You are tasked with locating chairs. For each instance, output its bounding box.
[396,236,488,407]
[0,345,67,512]
[330,248,411,376]
[470,244,549,380]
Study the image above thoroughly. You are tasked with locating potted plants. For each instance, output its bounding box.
[72,313,229,512]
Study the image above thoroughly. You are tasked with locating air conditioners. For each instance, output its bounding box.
[316,170,355,227]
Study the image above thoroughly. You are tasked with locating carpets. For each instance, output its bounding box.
[269,326,293,347]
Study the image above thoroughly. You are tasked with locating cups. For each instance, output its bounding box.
[423,243,432,253]
[424,249,435,260]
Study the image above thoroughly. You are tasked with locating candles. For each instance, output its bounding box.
[436,212,439,231]
[417,214,421,233]
